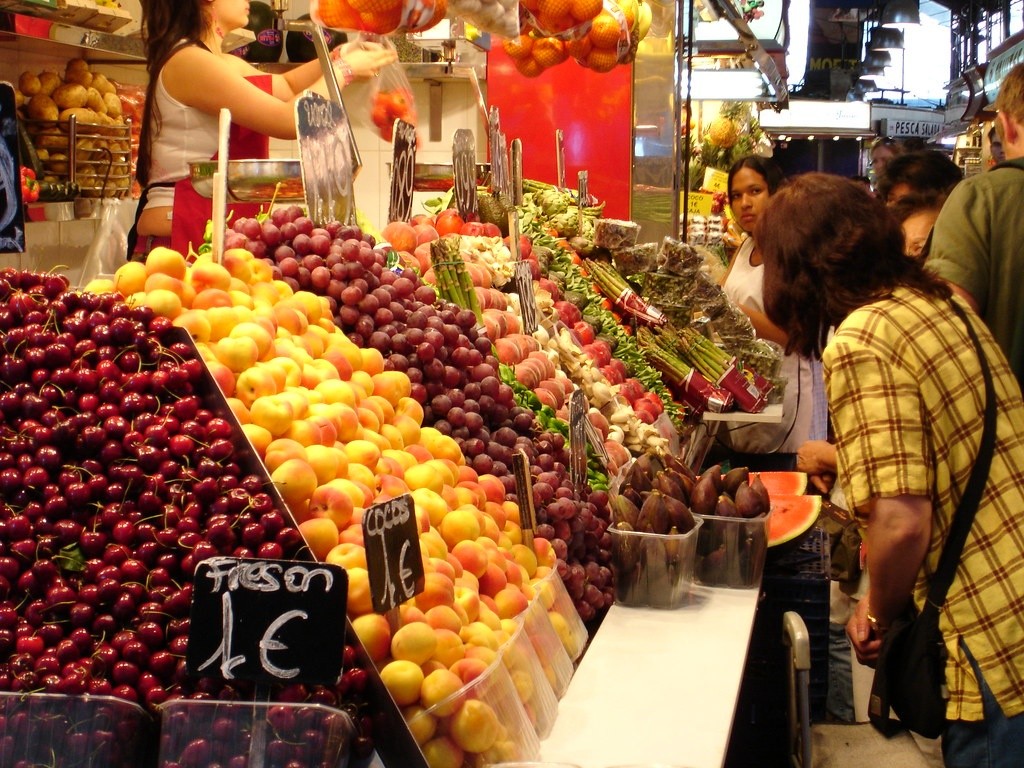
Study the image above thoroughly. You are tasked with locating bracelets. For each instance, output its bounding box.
[333,44,343,61]
[866,613,889,632]
[335,59,354,87]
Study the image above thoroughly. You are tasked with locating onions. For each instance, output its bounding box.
[435,209,532,260]
[519,257,664,425]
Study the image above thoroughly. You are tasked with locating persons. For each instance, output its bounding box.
[756,173,1024,767]
[848,134,965,207]
[127,0,399,267]
[922,61,1024,399]
[717,155,813,454]
[796,186,948,629]
[987,127,1006,162]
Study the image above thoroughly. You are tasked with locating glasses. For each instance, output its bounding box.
[881,136,902,153]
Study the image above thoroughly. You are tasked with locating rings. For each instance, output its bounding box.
[374,73,379,77]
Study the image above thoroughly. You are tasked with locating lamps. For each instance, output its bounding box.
[845,0,921,105]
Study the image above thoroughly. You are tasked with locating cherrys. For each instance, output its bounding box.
[0,268,373,768]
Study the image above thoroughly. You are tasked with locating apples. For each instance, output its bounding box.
[81,247,577,765]
[316,0,636,143]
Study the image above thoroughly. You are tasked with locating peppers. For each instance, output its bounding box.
[497,363,608,493]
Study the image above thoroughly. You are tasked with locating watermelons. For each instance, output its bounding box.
[695,471,822,548]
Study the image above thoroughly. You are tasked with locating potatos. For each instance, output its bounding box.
[12,59,128,216]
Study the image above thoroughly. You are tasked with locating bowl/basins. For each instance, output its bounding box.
[187,157,305,203]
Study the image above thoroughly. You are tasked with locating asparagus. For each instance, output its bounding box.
[523,178,604,220]
[429,237,482,327]
[584,252,733,385]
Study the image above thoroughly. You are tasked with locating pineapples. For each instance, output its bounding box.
[711,121,740,147]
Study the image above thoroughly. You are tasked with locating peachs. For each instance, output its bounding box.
[381,222,438,287]
[464,262,628,477]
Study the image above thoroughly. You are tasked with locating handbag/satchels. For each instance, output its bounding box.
[867,595,951,739]
